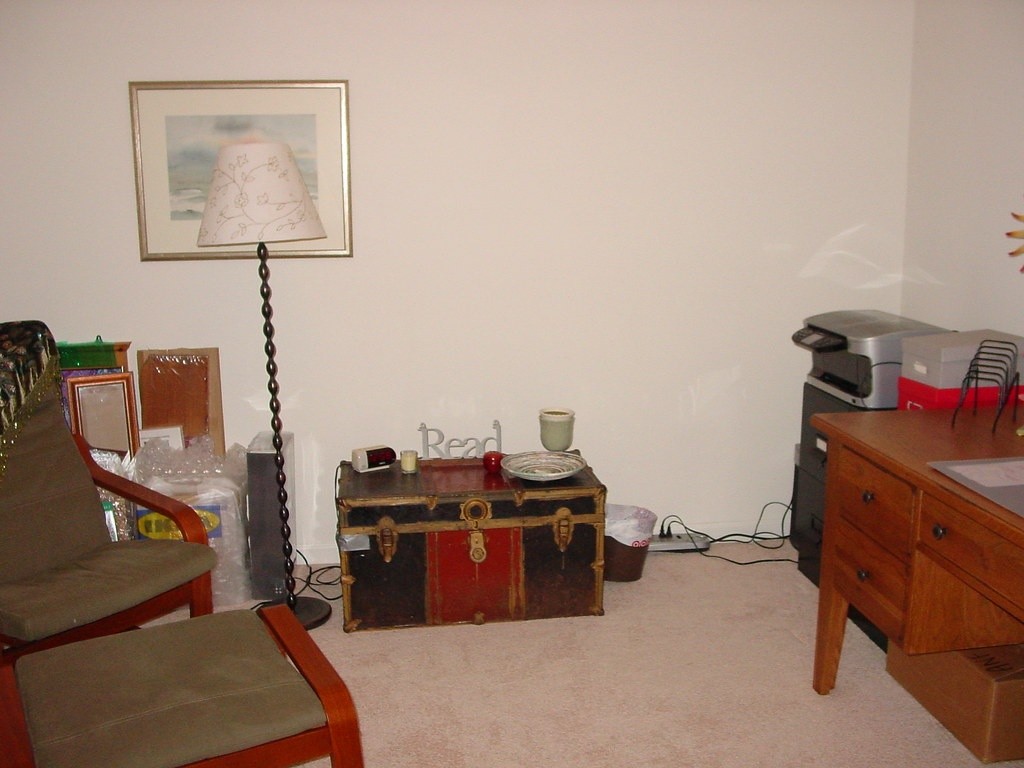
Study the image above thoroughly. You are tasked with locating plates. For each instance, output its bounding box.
[500,451,587,481]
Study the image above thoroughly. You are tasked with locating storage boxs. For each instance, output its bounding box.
[337,446,610,634]
[246,433,298,601]
[883,638,1024,764]
[898,329,1024,412]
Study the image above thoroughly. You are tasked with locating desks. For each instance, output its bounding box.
[804,410,1024,696]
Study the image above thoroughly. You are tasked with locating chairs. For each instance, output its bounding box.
[138,347,223,463]
[66,372,140,462]
[0,318,211,656]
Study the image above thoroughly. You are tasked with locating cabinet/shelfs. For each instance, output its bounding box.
[788,382,884,652]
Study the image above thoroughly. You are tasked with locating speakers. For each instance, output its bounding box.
[245,429,299,598]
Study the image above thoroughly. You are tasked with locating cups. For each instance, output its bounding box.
[539,407,575,451]
[400,450,417,475]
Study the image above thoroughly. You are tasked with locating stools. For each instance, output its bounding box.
[0,606,361,768]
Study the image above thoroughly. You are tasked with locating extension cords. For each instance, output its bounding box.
[649,533,710,551]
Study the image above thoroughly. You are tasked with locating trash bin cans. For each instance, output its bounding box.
[604,502,658,583]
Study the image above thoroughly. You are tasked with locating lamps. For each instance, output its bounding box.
[197,143,330,628]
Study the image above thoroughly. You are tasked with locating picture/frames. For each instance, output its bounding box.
[129,80,352,260]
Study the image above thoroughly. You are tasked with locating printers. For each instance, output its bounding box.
[791,309,951,409]
